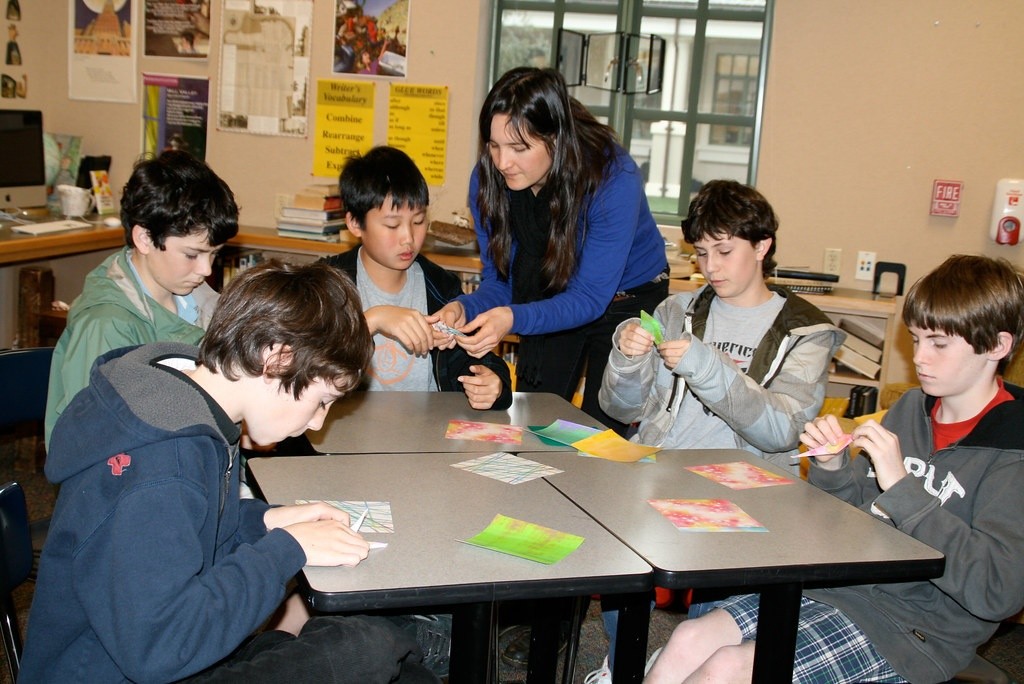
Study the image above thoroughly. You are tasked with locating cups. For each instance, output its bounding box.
[56,184,96,219]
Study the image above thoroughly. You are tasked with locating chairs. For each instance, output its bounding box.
[0,345,54,684]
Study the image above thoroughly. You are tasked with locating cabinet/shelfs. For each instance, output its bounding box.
[204,223,1023,423]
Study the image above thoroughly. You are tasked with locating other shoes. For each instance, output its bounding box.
[411,615,452,678]
[501,629,568,668]
[583,655,613,684]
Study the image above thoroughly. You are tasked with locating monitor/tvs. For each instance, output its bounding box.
[0,109,47,213]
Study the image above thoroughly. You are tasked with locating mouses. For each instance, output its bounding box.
[104,217,122,226]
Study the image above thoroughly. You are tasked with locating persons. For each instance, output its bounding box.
[429,66,671,670]
[582,179,1024,684]
[18,145,512,684]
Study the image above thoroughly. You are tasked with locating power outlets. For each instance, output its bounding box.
[823,247,842,275]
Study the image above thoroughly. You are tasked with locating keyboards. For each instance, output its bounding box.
[11,220,93,236]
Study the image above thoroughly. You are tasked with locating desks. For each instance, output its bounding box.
[1,206,128,263]
[246,391,945,684]
